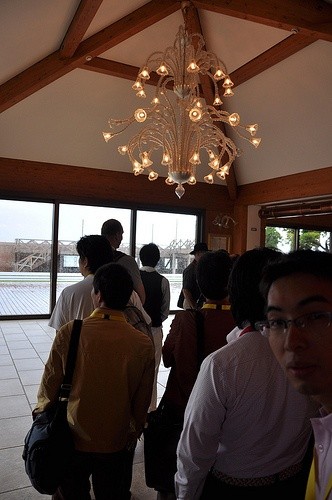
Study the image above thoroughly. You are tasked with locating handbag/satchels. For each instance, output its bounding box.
[145,407,185,487]
[23,410,81,494]
[123,305,153,341]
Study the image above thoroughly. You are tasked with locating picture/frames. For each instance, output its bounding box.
[208,232,231,253]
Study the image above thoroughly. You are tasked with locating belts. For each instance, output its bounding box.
[210,465,299,488]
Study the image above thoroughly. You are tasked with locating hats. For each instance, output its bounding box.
[190,242,211,254]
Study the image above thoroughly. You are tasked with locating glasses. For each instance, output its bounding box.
[255,312,332,338]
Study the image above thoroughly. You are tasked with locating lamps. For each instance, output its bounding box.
[213,215,240,232]
[102,8,262,199]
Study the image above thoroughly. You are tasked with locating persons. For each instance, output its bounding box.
[173,246,323,500]
[255,249,332,500]
[156,240,242,500]
[37,263,155,500]
[101,219,146,307]
[47,235,152,336]
[140,243,170,433]
